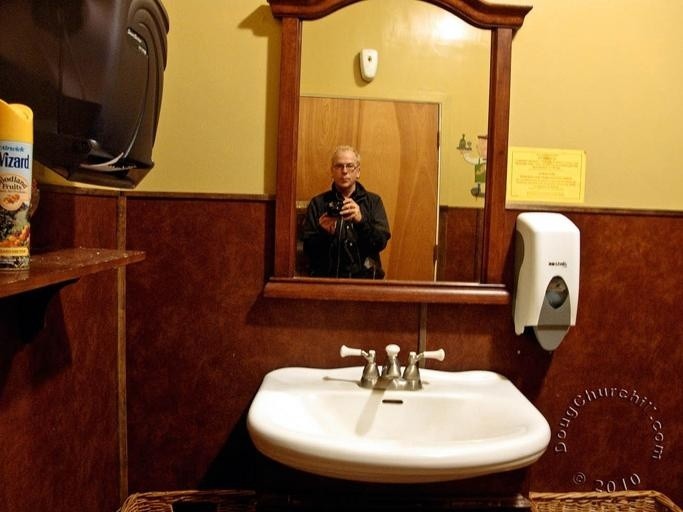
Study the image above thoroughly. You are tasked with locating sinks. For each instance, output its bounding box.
[248,365,555,486]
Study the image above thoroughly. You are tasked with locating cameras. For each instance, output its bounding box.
[327,200,344,217]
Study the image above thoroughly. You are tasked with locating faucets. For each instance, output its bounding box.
[379,344,408,393]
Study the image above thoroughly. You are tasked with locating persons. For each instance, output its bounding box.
[297,144,392,282]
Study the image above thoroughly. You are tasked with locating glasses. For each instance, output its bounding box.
[333,163,360,169]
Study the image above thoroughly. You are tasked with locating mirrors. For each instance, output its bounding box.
[267,0,532,309]
